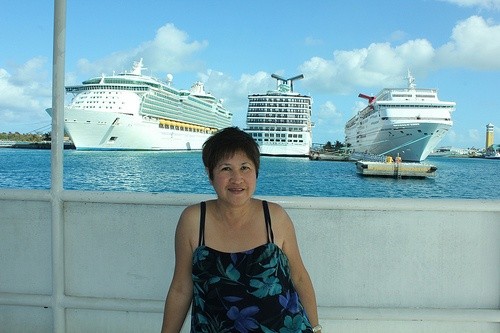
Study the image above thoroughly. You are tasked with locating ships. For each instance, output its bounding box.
[343,69,456,162]
[243,73,315,156]
[45,58,234,149]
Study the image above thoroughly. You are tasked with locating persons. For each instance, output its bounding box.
[385,152,402,163]
[161,127,324,333]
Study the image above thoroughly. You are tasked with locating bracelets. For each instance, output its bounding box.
[310,325,322,333]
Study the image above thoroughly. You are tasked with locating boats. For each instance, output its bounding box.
[355,161,439,176]
[309,141,352,161]
